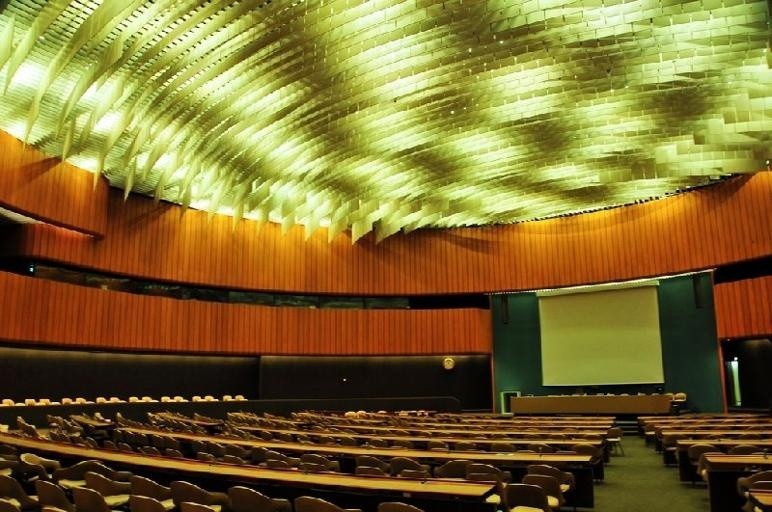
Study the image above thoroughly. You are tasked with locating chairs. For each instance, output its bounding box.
[0,388,514,512]
[514,387,771,511]
